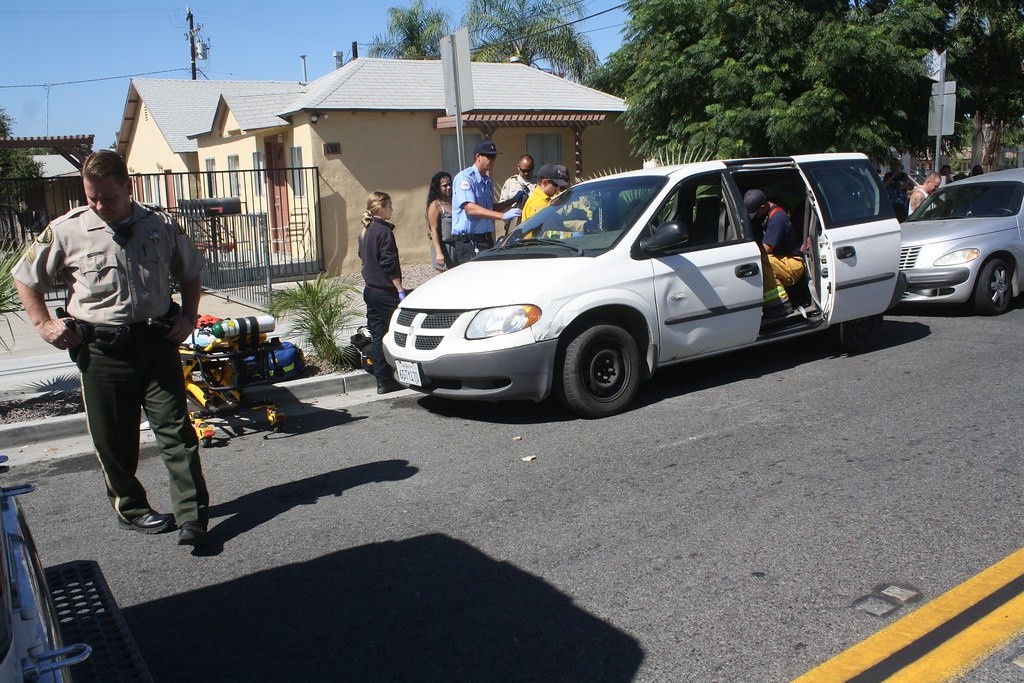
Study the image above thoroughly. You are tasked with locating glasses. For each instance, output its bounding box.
[518,166,534,173]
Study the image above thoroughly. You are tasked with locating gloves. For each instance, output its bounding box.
[503,208,522,220]
[514,191,525,202]
[399,292,405,301]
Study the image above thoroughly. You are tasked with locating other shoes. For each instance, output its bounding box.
[377,380,403,394]
[763,301,794,319]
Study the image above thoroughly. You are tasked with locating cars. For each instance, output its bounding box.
[896,167,1024,317]
[0,453,96,682]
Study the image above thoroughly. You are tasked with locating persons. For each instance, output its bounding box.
[745,189,806,313]
[358,191,406,395]
[425,171,452,275]
[521,163,593,238]
[452,140,521,265]
[12,152,210,548]
[885,157,914,222]
[498,154,537,230]
[908,172,941,218]
[940,164,987,183]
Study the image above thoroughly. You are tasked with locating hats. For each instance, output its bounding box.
[473,140,498,154]
[743,189,766,220]
[538,164,568,186]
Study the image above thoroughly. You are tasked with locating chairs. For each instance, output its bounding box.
[691,197,720,237]
[621,200,659,232]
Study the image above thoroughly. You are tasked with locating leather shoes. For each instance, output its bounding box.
[178,521,209,545]
[119,509,173,534]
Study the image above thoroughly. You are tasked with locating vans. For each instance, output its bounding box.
[382,149,907,418]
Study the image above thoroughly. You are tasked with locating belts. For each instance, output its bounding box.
[474,232,491,240]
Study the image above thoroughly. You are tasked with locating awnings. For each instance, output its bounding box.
[27,155,81,177]
